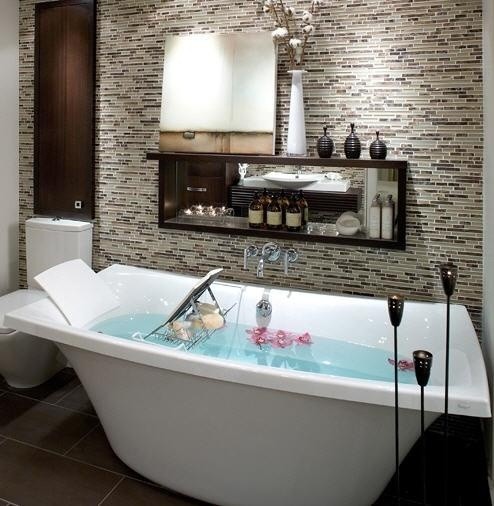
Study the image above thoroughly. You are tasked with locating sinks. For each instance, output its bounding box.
[264,172,318,187]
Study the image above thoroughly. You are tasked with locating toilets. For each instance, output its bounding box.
[0,218,93,388]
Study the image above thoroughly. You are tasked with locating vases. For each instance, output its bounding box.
[286,69,308,155]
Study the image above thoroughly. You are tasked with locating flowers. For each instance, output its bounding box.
[263,0,316,70]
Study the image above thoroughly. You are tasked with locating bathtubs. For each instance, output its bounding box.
[4,264,492,506]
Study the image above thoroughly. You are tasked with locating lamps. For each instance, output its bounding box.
[413,351,432,505]
[438,264,458,506]
[389,298,404,505]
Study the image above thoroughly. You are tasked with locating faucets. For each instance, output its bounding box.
[256,249,271,278]
